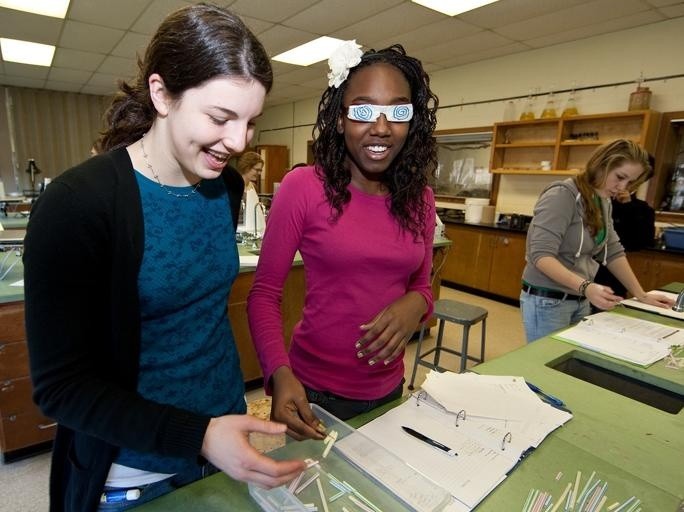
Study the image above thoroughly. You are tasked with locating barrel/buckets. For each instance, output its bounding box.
[465,198,490,224]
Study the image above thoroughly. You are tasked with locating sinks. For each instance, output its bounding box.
[249,250,263,257]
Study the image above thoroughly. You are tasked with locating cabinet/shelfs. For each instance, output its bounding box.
[489,109,660,178]
[0,265,308,454]
[438,224,683,304]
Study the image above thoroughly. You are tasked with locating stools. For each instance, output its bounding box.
[408,298,488,391]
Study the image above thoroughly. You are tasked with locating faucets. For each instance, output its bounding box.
[253,201,267,234]
[29,163,36,199]
[240,200,247,211]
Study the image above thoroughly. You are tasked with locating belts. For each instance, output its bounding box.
[520,284,587,302]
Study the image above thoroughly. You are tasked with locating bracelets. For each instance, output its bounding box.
[578,280,592,296]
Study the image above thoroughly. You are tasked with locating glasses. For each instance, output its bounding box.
[252,167,262,173]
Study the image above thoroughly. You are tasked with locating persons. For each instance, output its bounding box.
[519,134,676,348]
[591,151,653,316]
[89,138,104,159]
[237,151,266,239]
[245,41,440,440]
[22,4,308,512]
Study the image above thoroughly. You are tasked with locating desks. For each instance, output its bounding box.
[128,281,683,512]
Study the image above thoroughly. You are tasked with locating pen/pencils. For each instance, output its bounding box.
[526,380,563,407]
[402,425,458,456]
